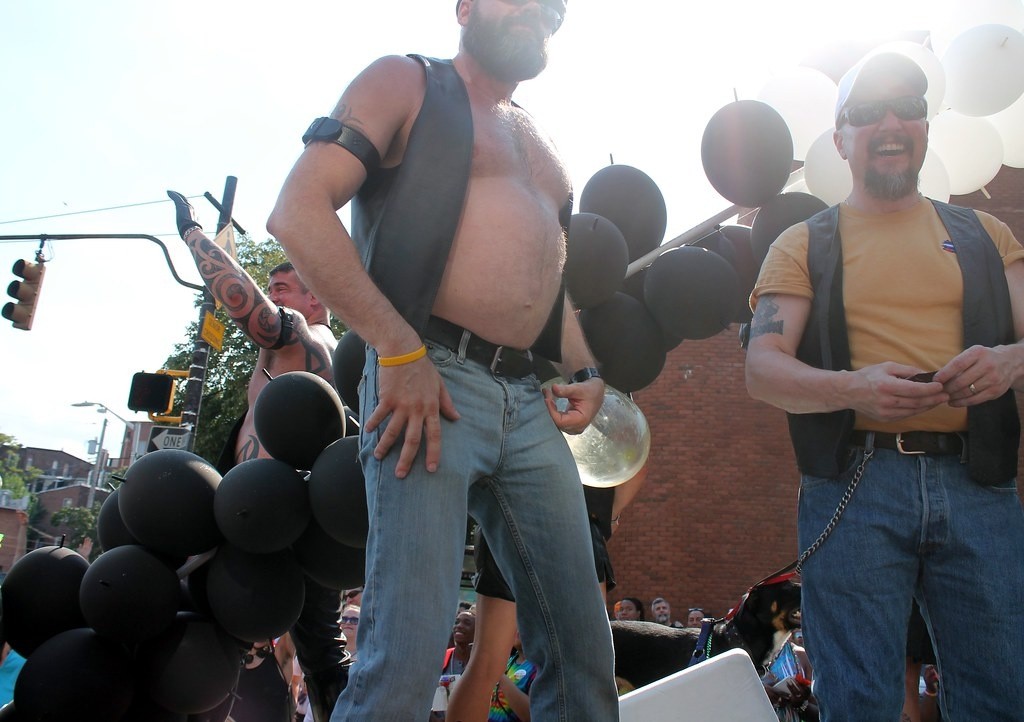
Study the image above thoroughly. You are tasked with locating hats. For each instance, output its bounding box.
[837,40,946,116]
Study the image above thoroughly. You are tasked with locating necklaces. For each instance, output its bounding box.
[313,322,332,330]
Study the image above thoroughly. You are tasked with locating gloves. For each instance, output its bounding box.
[168,190,202,239]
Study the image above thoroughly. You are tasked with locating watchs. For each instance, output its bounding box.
[611,515,621,525]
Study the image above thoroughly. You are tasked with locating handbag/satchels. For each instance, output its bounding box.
[432,673,462,711]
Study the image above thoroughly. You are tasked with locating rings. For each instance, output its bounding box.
[778,697,781,703]
[774,703,780,707]
[796,694,801,697]
[970,384,977,395]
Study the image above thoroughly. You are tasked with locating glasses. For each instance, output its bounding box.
[342,616,360,625]
[244,645,270,663]
[836,97,928,131]
[688,608,704,612]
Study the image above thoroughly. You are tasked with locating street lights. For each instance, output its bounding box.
[70,400,142,468]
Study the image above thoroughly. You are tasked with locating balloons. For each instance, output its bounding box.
[762,26,1023,208]
[702,88,792,208]
[566,154,832,396]
[537,377,650,487]
[0,330,368,721]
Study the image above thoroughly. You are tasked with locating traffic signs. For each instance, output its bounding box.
[146,425,189,453]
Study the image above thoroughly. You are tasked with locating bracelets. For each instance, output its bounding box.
[184,226,200,239]
[925,691,937,696]
[378,345,426,366]
[568,368,600,383]
[795,700,808,712]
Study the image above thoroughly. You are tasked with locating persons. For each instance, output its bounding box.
[165,190,363,721]
[743,53,1024,721]
[903,601,942,722]
[430,458,649,722]
[265,0,605,722]
[763,641,819,722]
[614,597,706,628]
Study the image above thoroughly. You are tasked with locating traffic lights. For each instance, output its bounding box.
[128,374,177,414]
[0,256,47,333]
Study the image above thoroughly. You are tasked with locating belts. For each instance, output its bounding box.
[851,427,973,455]
[424,316,538,377]
